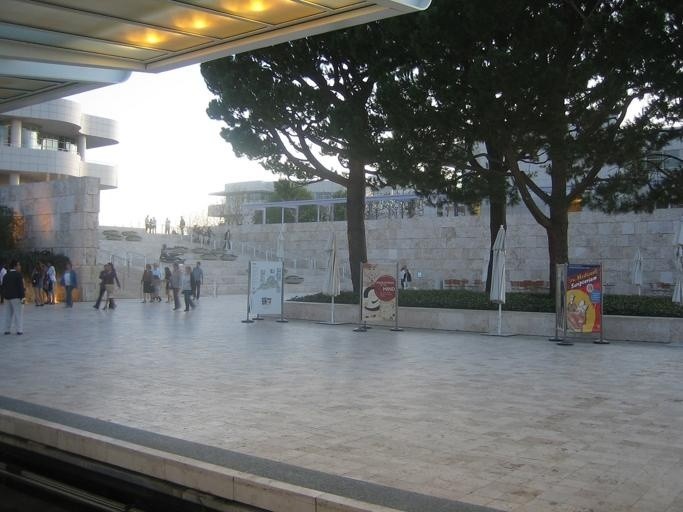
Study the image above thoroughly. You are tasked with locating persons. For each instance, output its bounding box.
[145,214,230,250]
[403,268,412,289]
[0,259,122,336]
[141,261,202,311]
[400,267,406,289]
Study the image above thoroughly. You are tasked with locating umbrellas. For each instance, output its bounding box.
[322,230,341,323]
[489,224,507,334]
[276,232,285,261]
[634,248,642,296]
[672,216,683,306]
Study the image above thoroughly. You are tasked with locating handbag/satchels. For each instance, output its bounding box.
[32,275,39,285]
[43,279,51,290]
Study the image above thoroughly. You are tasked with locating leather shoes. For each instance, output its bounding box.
[35,302,74,307]
[5,331,23,335]
[173,304,195,311]
[92,304,116,309]
